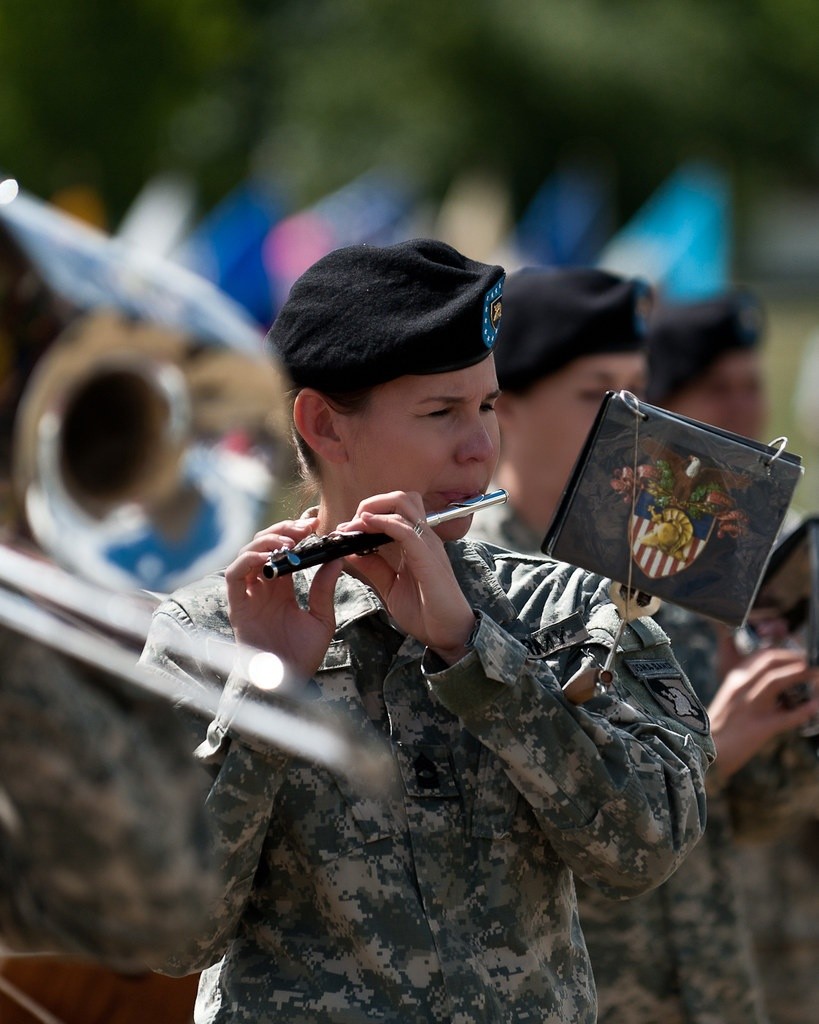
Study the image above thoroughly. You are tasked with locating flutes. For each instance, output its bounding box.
[262,488,510,582]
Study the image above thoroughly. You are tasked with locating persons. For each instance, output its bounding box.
[0,222,819,1024]
[130,236,717,1021]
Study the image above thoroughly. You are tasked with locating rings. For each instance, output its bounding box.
[413,526,424,536]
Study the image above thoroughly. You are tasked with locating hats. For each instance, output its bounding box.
[267,237,506,386]
[494,267,645,395]
[648,286,766,403]
